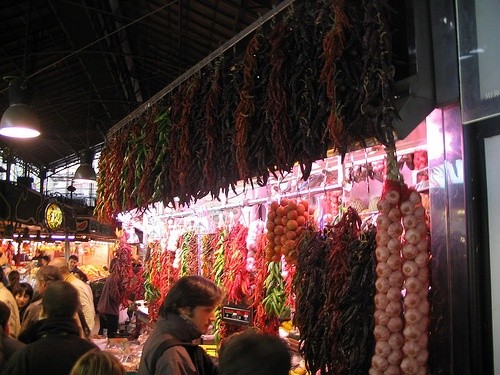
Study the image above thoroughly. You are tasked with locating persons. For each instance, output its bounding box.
[218,333,293,375]
[0,245,142,339]
[70,349,126,375]
[138,276,222,375]
[0,301,27,375]
[0,281,101,375]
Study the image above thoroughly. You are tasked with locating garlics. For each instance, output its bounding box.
[369,188,430,374]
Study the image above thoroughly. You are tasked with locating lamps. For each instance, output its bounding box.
[73,148,97,181]
[0,76,42,141]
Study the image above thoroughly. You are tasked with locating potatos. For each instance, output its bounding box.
[264,198,314,267]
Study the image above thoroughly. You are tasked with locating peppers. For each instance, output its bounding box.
[92,0,401,224]
[177,224,249,356]
[248,233,296,336]
[292,207,376,375]
[112,240,177,324]
